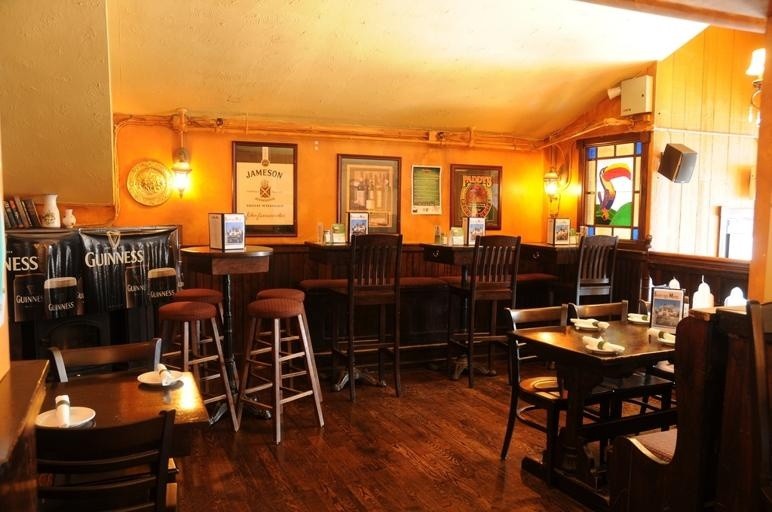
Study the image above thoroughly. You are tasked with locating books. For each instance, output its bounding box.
[3,194,43,231]
[344,211,369,243]
[546,217,571,246]
[207,213,246,250]
[460,216,486,246]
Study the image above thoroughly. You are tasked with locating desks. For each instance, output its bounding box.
[177,244,274,426]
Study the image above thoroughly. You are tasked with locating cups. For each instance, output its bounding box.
[64,208,76,230]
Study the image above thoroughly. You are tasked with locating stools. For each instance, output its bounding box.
[154,288,325,446]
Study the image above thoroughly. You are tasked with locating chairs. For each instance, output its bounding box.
[447,235,522,388]
[549,233,619,322]
[330,231,401,400]
[746,299,772,511]
[35,337,180,512]
[503,296,691,487]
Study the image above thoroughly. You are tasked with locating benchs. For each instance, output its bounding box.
[297,272,559,350]
[612,317,721,511]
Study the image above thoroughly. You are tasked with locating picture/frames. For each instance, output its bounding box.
[230,140,299,238]
[448,163,501,231]
[336,153,401,242]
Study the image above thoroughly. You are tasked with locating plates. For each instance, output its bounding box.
[134,369,180,388]
[36,405,98,432]
[568,302,691,359]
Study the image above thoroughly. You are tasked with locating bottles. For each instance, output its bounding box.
[568,223,588,247]
[312,217,335,245]
[433,224,449,248]
